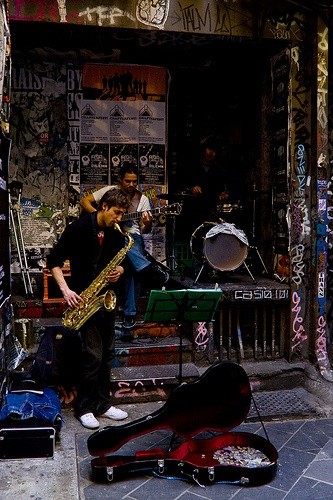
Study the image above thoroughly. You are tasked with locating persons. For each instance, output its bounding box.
[45,189,129,430]
[80,162,170,329]
[186,132,232,279]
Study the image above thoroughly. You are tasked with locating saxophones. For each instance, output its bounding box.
[60,220,135,332]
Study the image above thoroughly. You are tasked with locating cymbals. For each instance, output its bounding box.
[155,192,204,201]
[230,189,271,201]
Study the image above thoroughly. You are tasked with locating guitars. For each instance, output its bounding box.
[121,202,182,222]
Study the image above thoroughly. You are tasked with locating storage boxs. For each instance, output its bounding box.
[0,415,58,460]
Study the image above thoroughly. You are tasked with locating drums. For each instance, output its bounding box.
[189,220,250,273]
[213,198,243,213]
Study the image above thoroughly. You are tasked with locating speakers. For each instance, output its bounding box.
[30,326,85,387]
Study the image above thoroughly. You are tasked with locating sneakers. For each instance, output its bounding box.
[123,316,136,327]
[78,413,99,428]
[154,265,169,283]
[99,405,128,421]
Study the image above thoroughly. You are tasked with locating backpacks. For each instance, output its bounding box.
[30,326,84,387]
